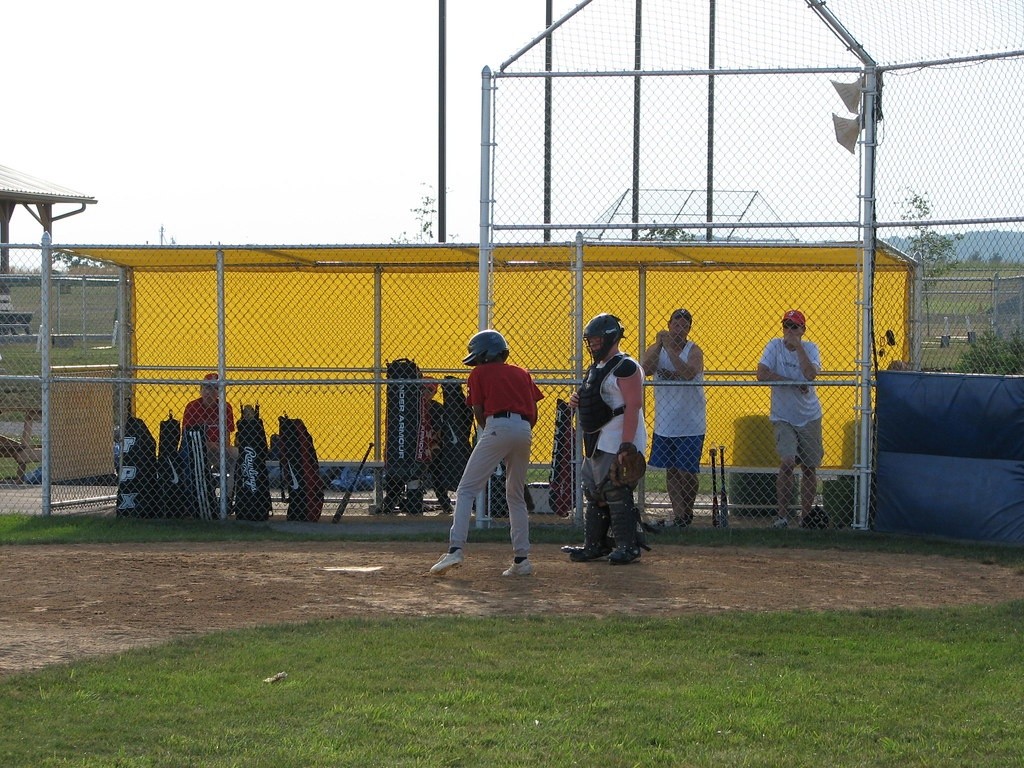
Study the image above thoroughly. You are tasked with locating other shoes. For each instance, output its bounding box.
[671,514,693,525]
[774,518,786,527]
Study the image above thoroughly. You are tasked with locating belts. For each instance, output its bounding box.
[493,411,531,421]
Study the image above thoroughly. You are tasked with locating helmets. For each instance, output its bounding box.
[583,312,625,363]
[462,328,509,365]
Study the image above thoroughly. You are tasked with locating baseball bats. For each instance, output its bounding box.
[709,449,720,527]
[719,445,730,527]
[331,442,375,524]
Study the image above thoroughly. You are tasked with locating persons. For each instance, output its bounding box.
[182,373,238,513]
[644,309,706,528]
[756,311,824,527]
[430,330,545,574]
[570,314,652,564]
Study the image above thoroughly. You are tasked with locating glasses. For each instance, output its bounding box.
[783,323,800,329]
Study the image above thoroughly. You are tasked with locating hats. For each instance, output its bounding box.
[781,310,806,327]
[203,373,219,380]
[670,309,691,321]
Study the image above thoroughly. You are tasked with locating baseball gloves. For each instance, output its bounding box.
[608,442,646,491]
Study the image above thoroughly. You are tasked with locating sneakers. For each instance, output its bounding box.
[606,545,641,564]
[502,558,532,577]
[430,548,463,577]
[569,537,613,561]
